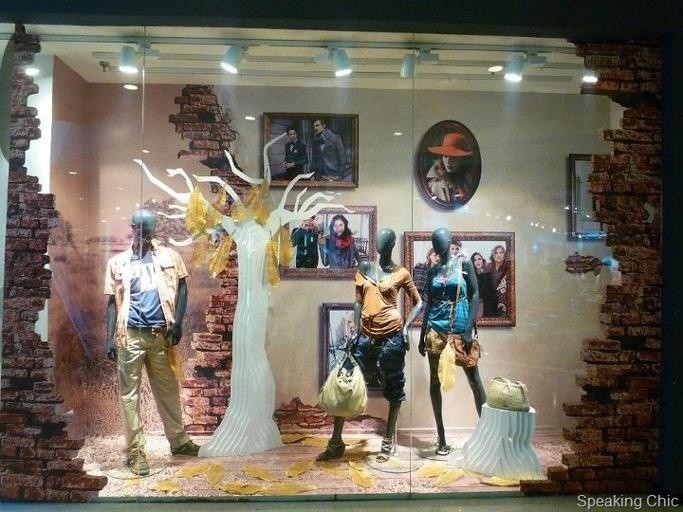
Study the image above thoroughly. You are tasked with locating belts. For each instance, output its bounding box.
[151,325,167,335]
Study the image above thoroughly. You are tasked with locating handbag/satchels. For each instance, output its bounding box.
[318,352,368,417]
[447,335,481,368]
[487,376,529,412]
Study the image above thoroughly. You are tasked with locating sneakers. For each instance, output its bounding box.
[171,440,199,456]
[127,449,150,475]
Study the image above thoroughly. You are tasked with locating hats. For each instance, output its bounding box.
[427,133,473,157]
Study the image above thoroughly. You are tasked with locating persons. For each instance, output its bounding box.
[290,215,324,268]
[316,229,424,461]
[318,215,362,269]
[282,127,308,180]
[104,209,200,475]
[413,241,508,317]
[311,118,345,181]
[424,133,475,204]
[419,228,488,455]
[329,312,383,387]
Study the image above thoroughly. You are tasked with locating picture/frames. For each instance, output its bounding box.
[262,112,360,190]
[418,119,482,211]
[278,204,378,279]
[568,153,608,241]
[403,231,516,327]
[320,302,383,391]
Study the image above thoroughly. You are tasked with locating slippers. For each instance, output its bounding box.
[436,445,449,455]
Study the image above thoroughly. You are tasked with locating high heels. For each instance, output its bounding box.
[376,440,392,462]
[316,441,345,461]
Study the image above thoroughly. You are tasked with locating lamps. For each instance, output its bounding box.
[118,46,139,75]
[503,55,525,82]
[220,45,242,75]
[332,49,352,77]
[400,54,415,80]
